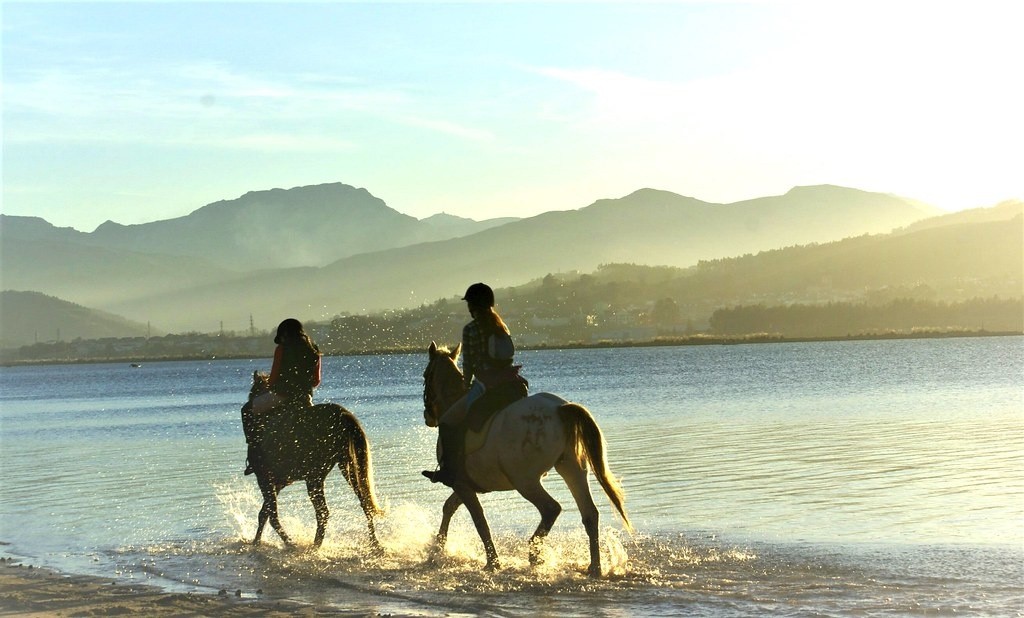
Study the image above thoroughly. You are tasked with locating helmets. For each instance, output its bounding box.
[461,283,495,307]
[274,319,303,344]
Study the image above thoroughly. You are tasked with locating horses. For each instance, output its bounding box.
[240,370,385,554]
[419,341,634,581]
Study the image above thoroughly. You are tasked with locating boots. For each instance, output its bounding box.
[242,418,265,475]
[421,426,459,488]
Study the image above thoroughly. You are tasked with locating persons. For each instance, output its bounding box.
[244,319,322,475]
[421,282,527,487]
